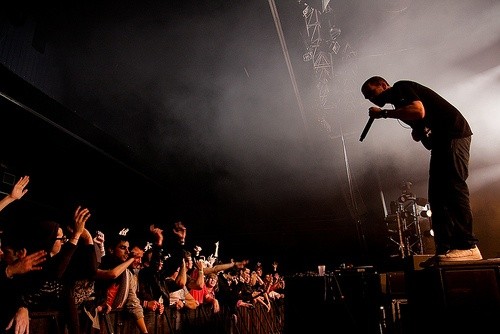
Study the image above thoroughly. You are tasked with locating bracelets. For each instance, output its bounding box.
[384,110,388,118]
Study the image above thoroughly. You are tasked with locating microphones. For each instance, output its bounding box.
[359,118,375,142]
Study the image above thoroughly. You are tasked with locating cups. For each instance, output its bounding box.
[318,265,326,276]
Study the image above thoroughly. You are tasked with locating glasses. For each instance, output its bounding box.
[55,236,67,242]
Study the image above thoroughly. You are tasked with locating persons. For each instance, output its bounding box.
[0,175,29,211]
[361,76,483,261]
[0,206,285,334]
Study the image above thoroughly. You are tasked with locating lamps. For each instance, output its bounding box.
[421,209,431,217]
[398,196,406,203]
[401,212,408,217]
[424,229,434,237]
[416,197,427,206]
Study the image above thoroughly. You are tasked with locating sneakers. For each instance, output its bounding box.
[439,246,483,261]
[418,254,440,267]
